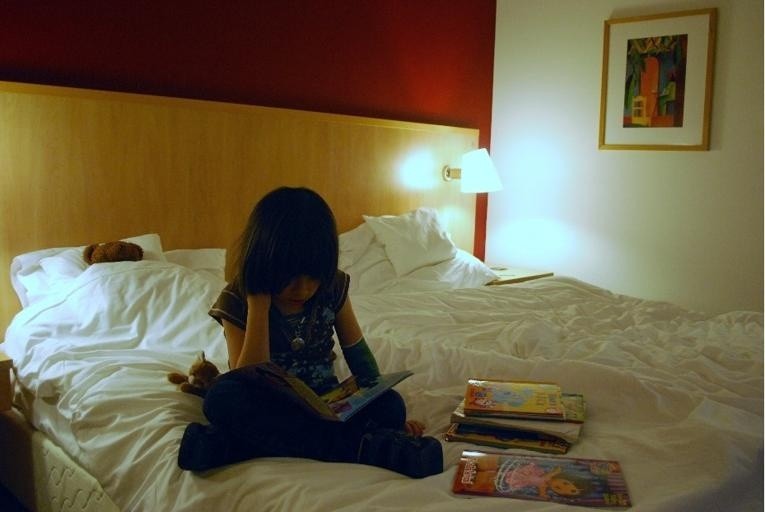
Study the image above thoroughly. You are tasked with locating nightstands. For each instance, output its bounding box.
[488,265,555,286]
[0,351,14,412]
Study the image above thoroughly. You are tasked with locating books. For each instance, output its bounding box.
[449,450,630,508]
[277,369,414,422]
[444,376,586,454]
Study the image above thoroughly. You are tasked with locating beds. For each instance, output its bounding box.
[0,82,764,510]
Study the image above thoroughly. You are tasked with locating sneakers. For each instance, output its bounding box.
[365,427,444,479]
[177,422,237,470]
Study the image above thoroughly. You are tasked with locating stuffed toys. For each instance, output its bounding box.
[82,240,144,265]
[168,349,219,396]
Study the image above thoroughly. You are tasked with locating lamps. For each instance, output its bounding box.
[442,147,502,194]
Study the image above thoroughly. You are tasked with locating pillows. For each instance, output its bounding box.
[39,233,169,287]
[360,207,457,278]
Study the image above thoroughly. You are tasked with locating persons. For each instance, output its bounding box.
[177,185,443,478]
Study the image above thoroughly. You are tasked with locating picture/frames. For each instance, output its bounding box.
[598,8,717,151]
